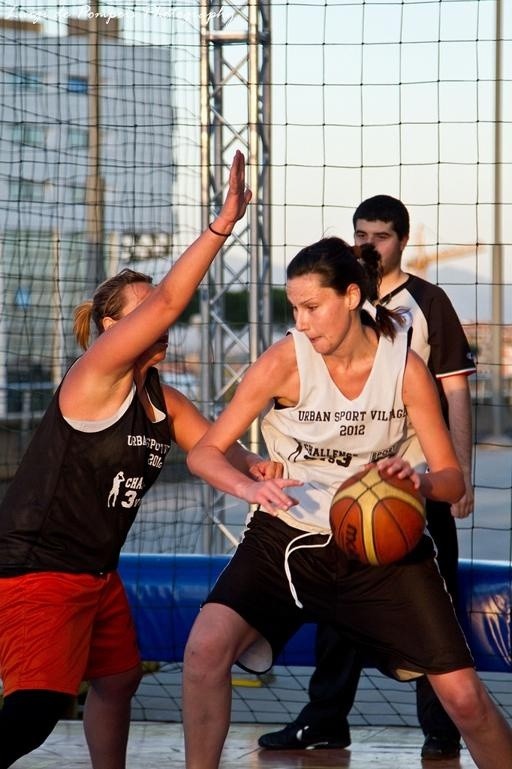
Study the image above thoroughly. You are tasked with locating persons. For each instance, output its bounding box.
[176,234,512,767]
[256,192,478,761]
[0,151,289,768]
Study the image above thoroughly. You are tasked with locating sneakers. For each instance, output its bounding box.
[257,701,351,750]
[420,730,460,760]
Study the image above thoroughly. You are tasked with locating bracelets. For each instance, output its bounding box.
[207,223,232,238]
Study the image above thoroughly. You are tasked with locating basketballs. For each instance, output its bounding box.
[329,468,426,565]
